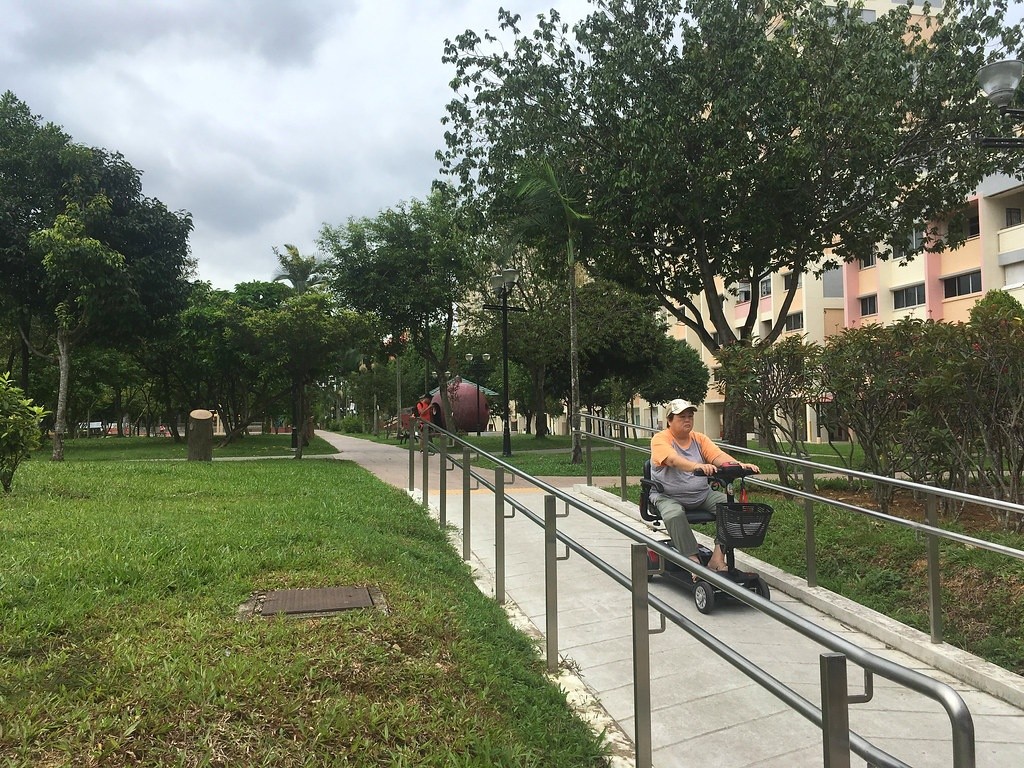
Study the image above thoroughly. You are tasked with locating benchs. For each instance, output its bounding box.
[399,430,418,445]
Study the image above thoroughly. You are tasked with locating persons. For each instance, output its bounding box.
[649,399,762,583]
[417,394,435,454]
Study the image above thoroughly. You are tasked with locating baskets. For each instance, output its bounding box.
[716,502,775,549]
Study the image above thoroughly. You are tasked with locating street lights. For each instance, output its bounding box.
[491,267,519,455]
[431,371,451,402]
[465,353,490,436]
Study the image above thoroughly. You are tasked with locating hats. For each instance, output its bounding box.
[666,398,698,419]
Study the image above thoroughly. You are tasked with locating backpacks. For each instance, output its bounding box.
[411,401,423,419]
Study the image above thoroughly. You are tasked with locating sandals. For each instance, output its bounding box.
[707,559,728,572]
[692,575,700,583]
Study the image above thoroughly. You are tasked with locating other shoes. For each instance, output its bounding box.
[420,450,434,454]
